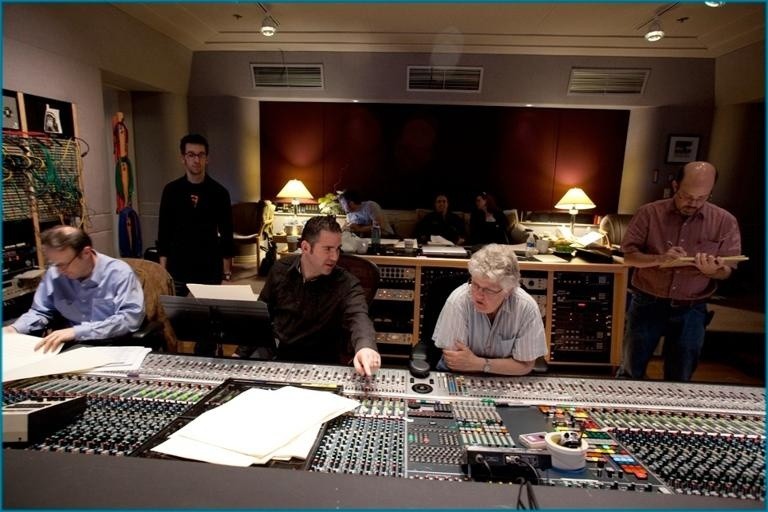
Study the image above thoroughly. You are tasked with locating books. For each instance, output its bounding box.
[659,253,749,269]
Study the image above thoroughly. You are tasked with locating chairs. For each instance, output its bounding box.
[421,275,482,369]
[334,252,382,299]
[229,201,269,276]
[106,257,178,347]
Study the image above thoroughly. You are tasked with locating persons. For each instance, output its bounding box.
[411,193,466,246]
[431,242,548,376]
[230,215,383,377]
[156,132,236,297]
[1,224,147,355]
[614,160,743,381]
[463,190,511,245]
[338,189,381,238]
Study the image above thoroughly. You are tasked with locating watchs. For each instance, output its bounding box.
[223,271,233,276]
[483,357,492,372]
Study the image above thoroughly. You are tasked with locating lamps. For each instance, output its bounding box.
[632,1,725,44]
[255,1,281,36]
[553,187,597,245]
[275,178,316,236]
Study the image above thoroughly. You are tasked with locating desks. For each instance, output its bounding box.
[273,234,303,260]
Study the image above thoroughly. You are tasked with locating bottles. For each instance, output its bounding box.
[370,222,381,245]
[597,209,603,225]
[525,232,535,257]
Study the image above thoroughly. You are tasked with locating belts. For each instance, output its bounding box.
[633,287,706,308]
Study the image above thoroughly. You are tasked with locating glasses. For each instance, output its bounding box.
[185,152,206,160]
[48,254,78,269]
[677,191,713,203]
[468,277,502,296]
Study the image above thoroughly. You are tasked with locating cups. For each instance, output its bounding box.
[287,236,298,252]
[537,239,548,254]
[404,239,414,253]
[283,224,303,235]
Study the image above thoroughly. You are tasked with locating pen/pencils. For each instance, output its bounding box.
[667,241,684,257]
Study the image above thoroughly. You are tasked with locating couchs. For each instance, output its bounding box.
[339,207,538,243]
[600,212,638,251]
